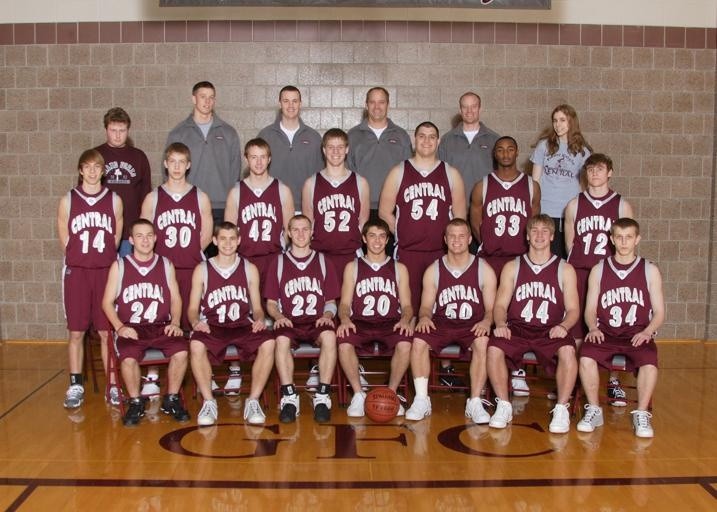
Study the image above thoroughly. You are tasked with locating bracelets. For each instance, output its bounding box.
[115,324,124,333]
[322,303,338,317]
[557,322,570,333]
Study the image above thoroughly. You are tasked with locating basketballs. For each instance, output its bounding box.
[364,387,399,423]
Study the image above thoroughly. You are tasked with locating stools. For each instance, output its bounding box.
[100,323,185,418]
[425,342,494,411]
[344,335,410,409]
[79,321,107,395]
[190,343,270,413]
[269,338,342,410]
[507,350,573,417]
[569,352,657,419]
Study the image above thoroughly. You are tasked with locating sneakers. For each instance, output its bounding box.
[195,398,218,425]
[63,383,84,408]
[606,378,627,407]
[196,365,245,396]
[508,370,577,400]
[631,409,654,438]
[548,403,570,434]
[313,382,335,422]
[242,398,266,426]
[140,376,161,402]
[392,397,404,416]
[439,364,460,392]
[489,399,515,429]
[348,363,370,392]
[102,383,128,407]
[464,397,491,426]
[160,393,192,422]
[119,398,145,427]
[576,404,604,433]
[404,394,432,421]
[279,384,302,423]
[303,365,326,391]
[345,390,369,417]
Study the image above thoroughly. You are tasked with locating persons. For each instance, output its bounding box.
[334,217,417,420]
[261,214,343,425]
[402,216,499,426]
[468,133,542,400]
[252,84,328,216]
[221,137,296,396]
[343,86,413,258]
[100,217,190,426]
[158,80,242,260]
[575,217,666,438]
[139,142,225,402]
[184,219,276,427]
[376,121,468,397]
[485,213,581,436]
[432,89,500,258]
[546,152,635,409]
[528,103,593,262]
[56,149,128,410]
[300,128,372,394]
[75,105,152,260]
